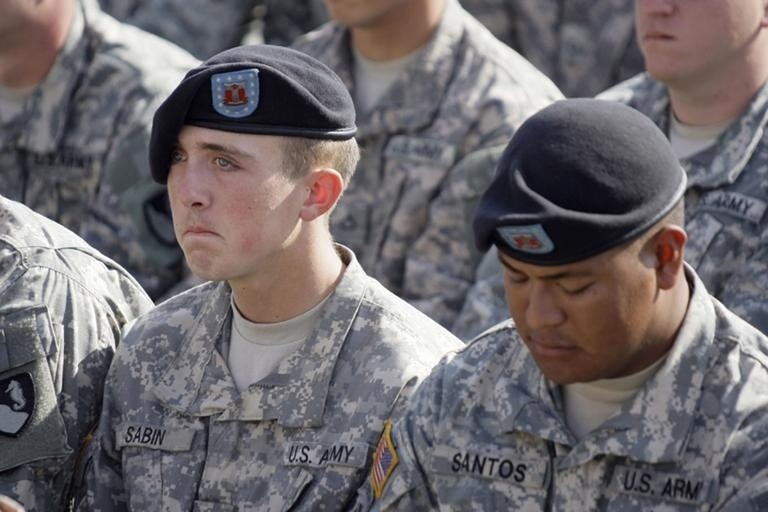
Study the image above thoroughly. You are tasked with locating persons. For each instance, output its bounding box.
[1,196,156,511]
[99,0,646,97]
[355,98,767,511]
[1,1,206,301]
[449,0,767,345]
[286,0,570,331]
[78,45,467,510]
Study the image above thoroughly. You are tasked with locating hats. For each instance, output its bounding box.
[473,98,687,267]
[149,45,358,186]
